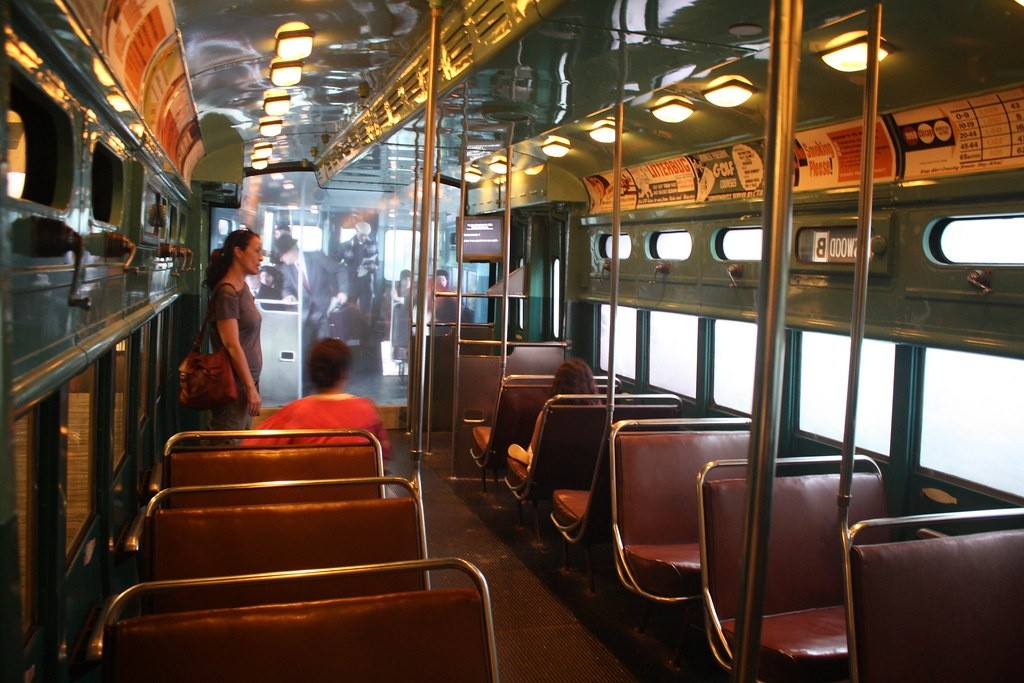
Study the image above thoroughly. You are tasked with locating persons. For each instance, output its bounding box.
[508,357,602,473]
[238,338,393,465]
[245,221,475,336]
[179,229,263,447]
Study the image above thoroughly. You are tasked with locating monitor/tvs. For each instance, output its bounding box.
[455,215,504,263]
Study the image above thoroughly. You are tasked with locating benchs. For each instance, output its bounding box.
[125,470,433,615]
[147,425,389,507]
[599,416,751,674]
[856,495,1024,683]
[689,454,882,683]
[536,416,750,594]
[71,552,500,683]
[468,370,623,509]
[497,393,685,548]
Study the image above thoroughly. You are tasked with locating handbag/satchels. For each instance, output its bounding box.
[178,347,242,408]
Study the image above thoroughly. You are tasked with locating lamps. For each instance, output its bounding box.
[701,79,761,108]
[588,124,616,144]
[251,158,268,171]
[259,120,283,137]
[466,170,483,183]
[650,98,698,125]
[254,143,275,159]
[539,142,572,159]
[267,62,305,87]
[261,96,292,116]
[488,160,513,176]
[816,33,900,74]
[273,29,315,62]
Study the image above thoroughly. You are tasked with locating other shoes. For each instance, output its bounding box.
[508,444,530,464]
